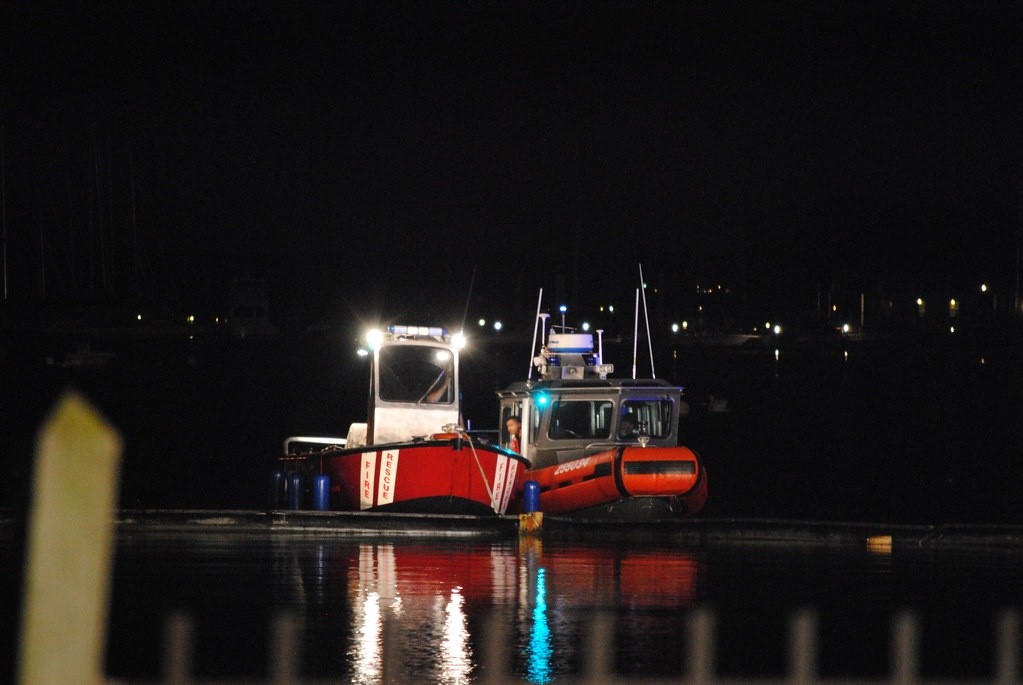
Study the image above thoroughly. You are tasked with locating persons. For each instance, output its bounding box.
[505,416,521,453]
[620,413,637,439]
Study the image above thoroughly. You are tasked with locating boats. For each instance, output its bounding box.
[277,325,533,530]
[481,263,709,531]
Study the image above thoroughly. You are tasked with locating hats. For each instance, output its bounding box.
[622,414,635,424]
